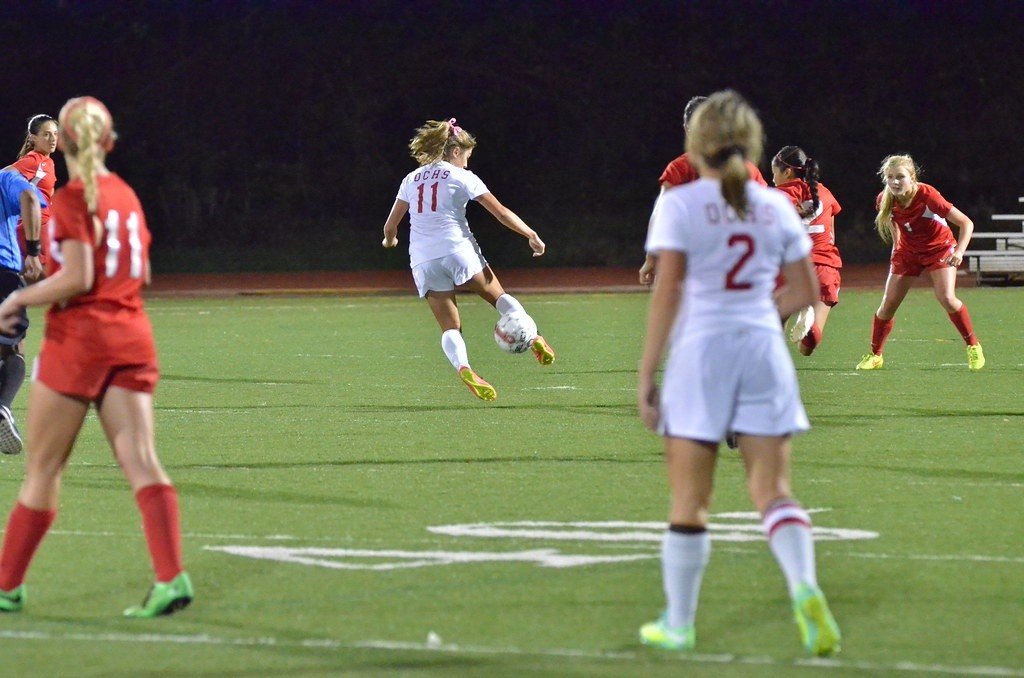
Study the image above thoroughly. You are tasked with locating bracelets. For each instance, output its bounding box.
[24,239,41,256]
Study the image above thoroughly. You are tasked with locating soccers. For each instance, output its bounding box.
[494,310,537,354]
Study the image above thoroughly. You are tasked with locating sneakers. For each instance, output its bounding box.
[531,335,555,365]
[122,572,193,618]
[639,616,695,650]
[794,586,841,658]
[790,305,814,343]
[0,583,26,611]
[856,351,883,370]
[0,405,23,454]
[967,342,985,369]
[458,364,497,402]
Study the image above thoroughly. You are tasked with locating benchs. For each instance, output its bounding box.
[964,195,1024,283]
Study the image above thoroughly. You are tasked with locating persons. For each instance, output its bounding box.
[637,91,843,658]
[4,114,59,286]
[639,97,768,289]
[0,169,47,455]
[0,96,196,619]
[382,117,555,401]
[772,146,842,356]
[857,155,985,371]
